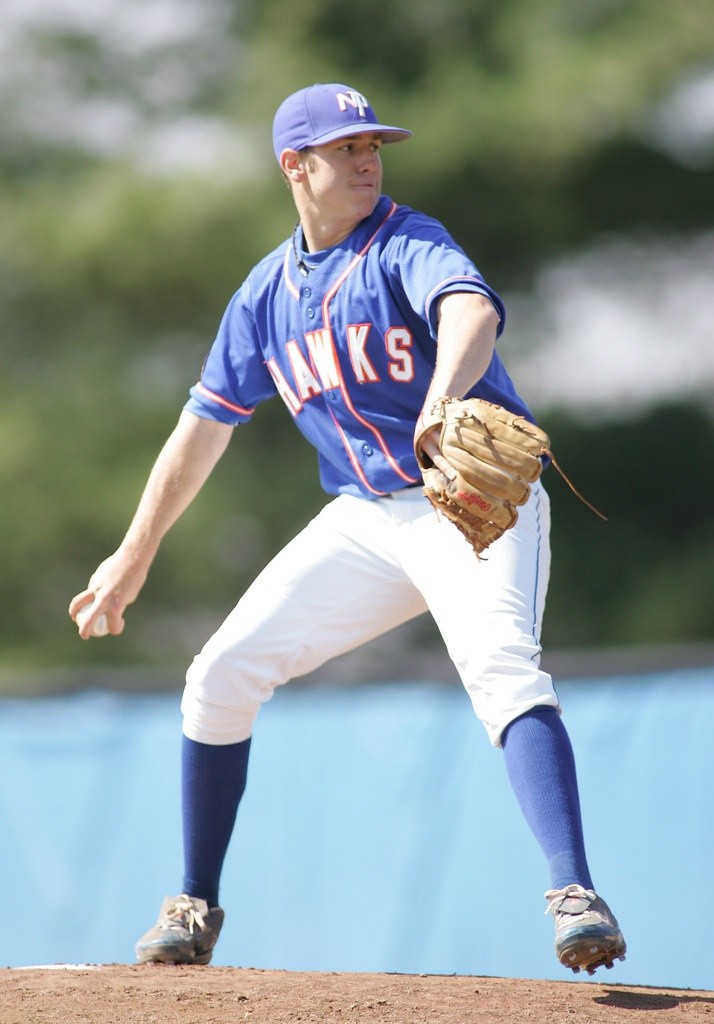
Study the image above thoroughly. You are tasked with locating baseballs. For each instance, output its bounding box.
[74,600,112,639]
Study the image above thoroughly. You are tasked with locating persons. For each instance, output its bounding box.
[67,82,627,976]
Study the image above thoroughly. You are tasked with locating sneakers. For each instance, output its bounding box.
[544,883,628,974]
[135,894,224,966]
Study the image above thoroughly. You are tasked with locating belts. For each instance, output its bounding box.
[402,481,424,490]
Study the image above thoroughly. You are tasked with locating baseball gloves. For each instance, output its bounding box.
[413,397,551,553]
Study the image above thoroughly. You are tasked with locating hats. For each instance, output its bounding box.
[271,83,413,166]
